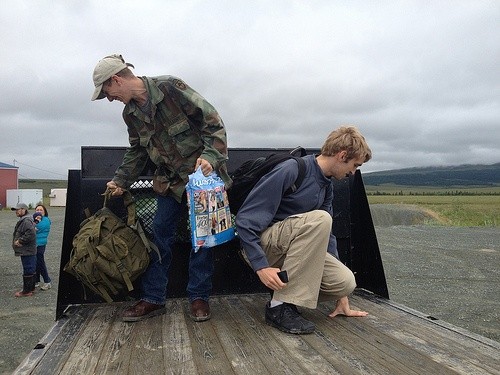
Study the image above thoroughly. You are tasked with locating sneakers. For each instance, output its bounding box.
[266,302,315,333]
[41,282,51,291]
[122,299,165,321]
[15,291,32,297]
[34,282,39,287]
[191,299,210,320]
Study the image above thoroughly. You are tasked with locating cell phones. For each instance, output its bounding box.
[277,271,288,283]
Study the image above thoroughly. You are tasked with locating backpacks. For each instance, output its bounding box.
[226,146,307,256]
[64,186,161,305]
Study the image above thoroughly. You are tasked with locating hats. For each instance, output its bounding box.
[33,212,42,219]
[11,203,28,211]
[91,54,134,101]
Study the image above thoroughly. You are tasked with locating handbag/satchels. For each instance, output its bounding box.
[185,165,235,253]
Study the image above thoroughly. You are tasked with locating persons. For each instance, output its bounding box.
[92,54,233,322]
[193,186,229,235]
[235,127,373,334]
[11,202,51,297]
